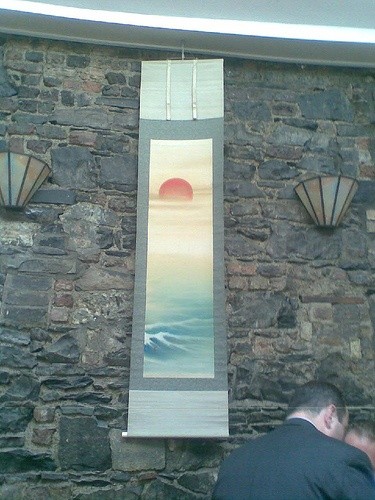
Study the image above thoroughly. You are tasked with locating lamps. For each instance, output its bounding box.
[292,174,360,231]
[0,149,54,213]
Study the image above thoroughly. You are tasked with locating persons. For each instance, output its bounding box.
[343,419,375,468]
[211,378,375,500]
